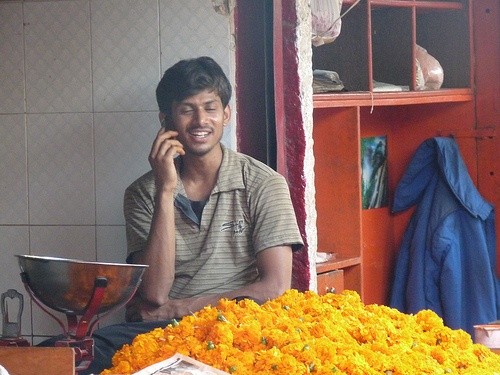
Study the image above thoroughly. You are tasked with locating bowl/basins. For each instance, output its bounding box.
[14,254,150,315]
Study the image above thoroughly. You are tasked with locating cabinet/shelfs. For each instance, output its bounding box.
[306,1,479,305]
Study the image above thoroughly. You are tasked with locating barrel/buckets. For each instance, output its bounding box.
[473,321,500,349]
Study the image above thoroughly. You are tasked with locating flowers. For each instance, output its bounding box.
[99,289,500,375]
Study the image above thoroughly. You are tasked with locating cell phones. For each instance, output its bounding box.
[161,116,181,159]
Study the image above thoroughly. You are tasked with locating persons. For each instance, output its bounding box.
[33,57,304,375]
